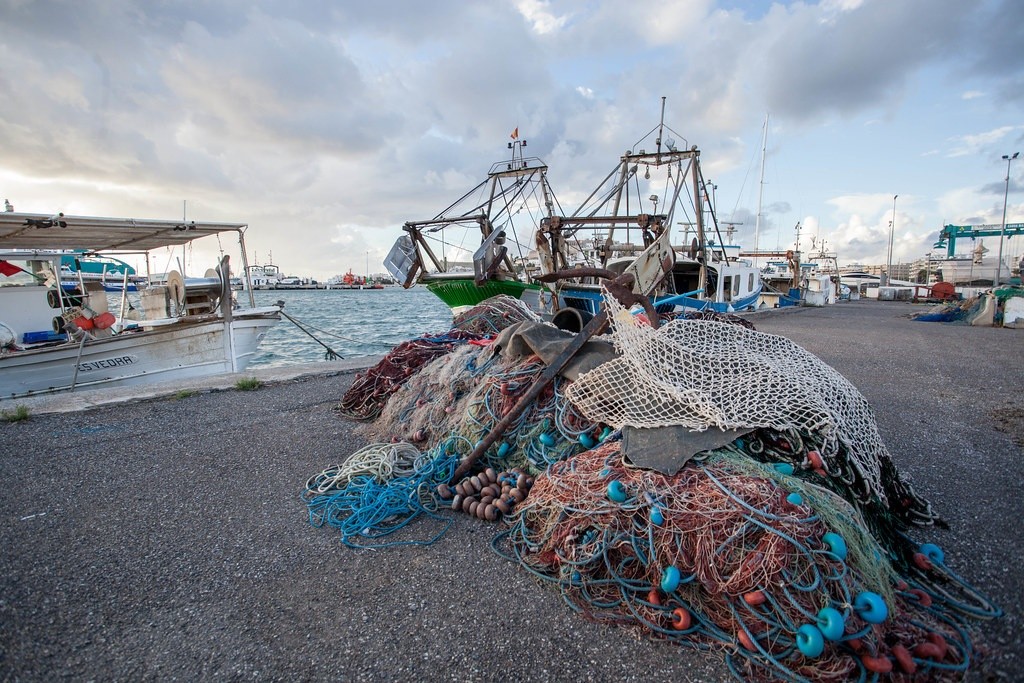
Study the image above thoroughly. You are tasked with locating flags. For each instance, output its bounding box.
[510,127,518,140]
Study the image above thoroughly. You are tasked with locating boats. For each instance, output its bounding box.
[1,199,285,400]
[230,250,383,289]
[383,97,850,312]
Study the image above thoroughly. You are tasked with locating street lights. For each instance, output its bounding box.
[993,152,1020,287]
[888,195,898,285]
